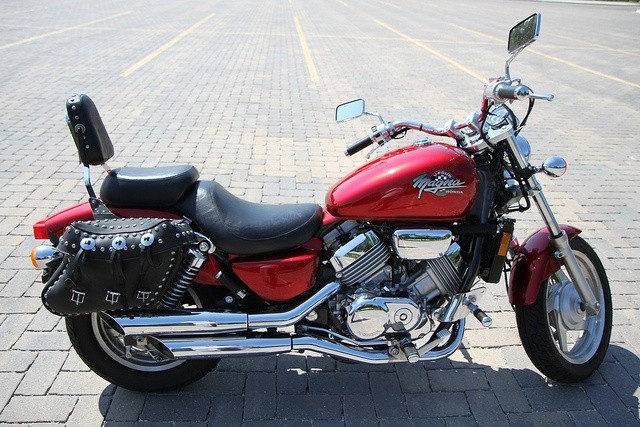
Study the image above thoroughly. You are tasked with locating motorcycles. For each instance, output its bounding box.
[31,12,613,393]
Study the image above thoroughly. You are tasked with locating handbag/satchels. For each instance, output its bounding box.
[42,219,195,316]
